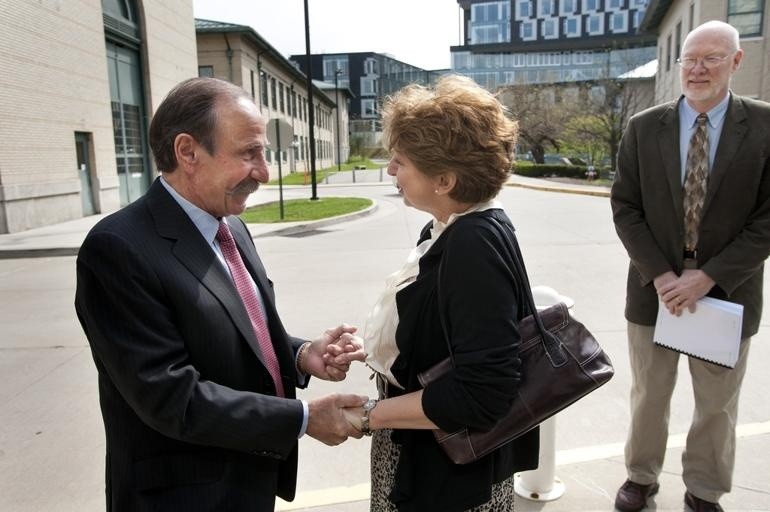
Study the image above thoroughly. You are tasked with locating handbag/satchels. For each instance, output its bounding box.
[414,212,615,466]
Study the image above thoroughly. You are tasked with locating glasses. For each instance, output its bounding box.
[676,52,731,68]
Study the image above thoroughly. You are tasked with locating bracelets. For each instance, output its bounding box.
[297,342,311,371]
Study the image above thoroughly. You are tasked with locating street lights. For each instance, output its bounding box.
[334,66,343,171]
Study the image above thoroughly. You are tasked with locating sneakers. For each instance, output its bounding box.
[615,477,660,512]
[684,489,724,512]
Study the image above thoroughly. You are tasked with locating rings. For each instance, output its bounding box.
[676,297,681,303]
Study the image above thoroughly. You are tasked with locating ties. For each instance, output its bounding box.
[215,221,286,400]
[681,114,711,251]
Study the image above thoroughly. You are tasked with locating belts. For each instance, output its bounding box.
[682,250,699,260]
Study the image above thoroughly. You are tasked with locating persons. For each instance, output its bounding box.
[610,20,770,512]
[75,77,369,512]
[334,75,540,512]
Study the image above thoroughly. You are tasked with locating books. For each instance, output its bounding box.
[652,294,744,369]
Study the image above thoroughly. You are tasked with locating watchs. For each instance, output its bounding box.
[361,399,377,435]
